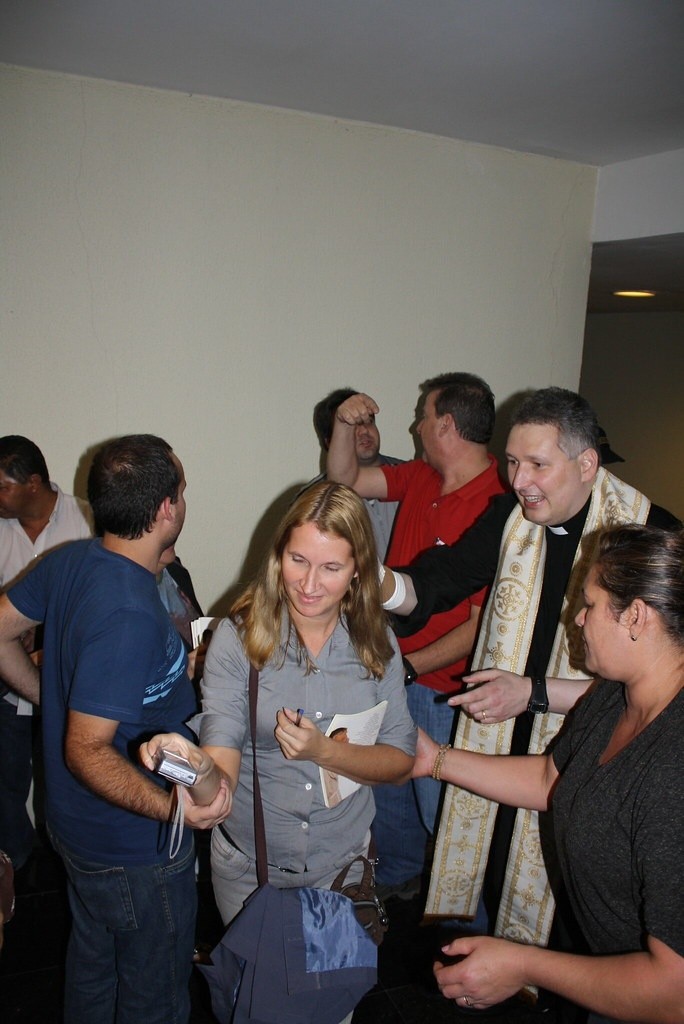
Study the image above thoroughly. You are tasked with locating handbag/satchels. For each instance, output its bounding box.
[331,856,385,947]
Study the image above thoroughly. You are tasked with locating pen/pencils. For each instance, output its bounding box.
[296,710,306,727]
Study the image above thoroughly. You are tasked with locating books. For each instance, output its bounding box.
[320,701,388,809]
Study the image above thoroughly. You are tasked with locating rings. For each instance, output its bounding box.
[482,711,488,721]
[464,997,471,1006]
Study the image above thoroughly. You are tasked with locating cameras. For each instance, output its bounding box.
[152,747,197,788]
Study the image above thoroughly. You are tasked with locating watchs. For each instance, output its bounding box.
[527,675,550,715]
[402,656,417,686]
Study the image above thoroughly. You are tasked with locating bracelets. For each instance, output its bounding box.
[432,743,450,781]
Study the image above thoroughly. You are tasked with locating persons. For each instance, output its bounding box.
[374,384,684,1016]
[0,434,234,1024]
[328,370,510,938]
[294,389,404,565]
[412,524,684,1024]
[139,481,417,1024]
